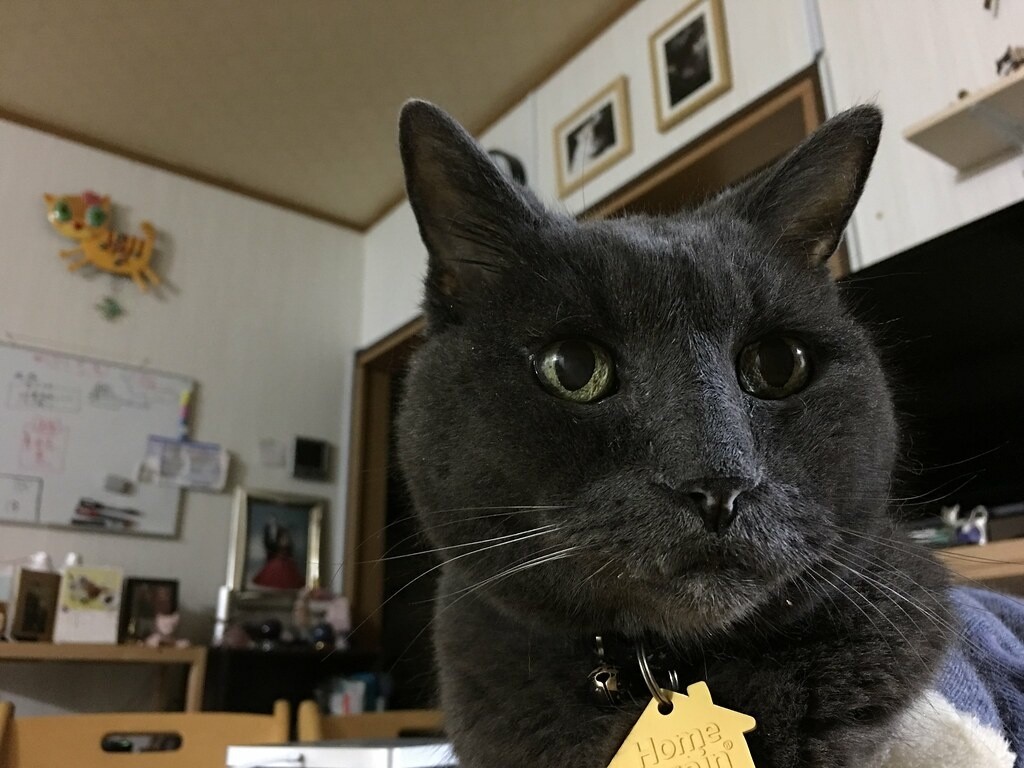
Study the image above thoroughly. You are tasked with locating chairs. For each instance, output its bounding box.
[0,700,443,768]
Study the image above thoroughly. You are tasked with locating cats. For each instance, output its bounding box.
[395,94,1024,768]
[42,189,163,293]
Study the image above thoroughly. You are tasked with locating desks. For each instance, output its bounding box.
[0,642,207,714]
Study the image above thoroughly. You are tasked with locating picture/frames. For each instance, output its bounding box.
[647,0,733,134]
[226,485,329,592]
[119,578,179,644]
[551,74,633,198]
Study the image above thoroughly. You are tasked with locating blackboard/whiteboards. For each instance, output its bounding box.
[1,330,201,541]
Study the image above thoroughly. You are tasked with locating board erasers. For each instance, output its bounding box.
[104,473,134,495]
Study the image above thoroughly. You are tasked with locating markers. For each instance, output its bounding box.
[71,496,141,529]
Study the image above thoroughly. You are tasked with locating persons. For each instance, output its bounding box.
[252,505,298,587]
[570,113,603,173]
